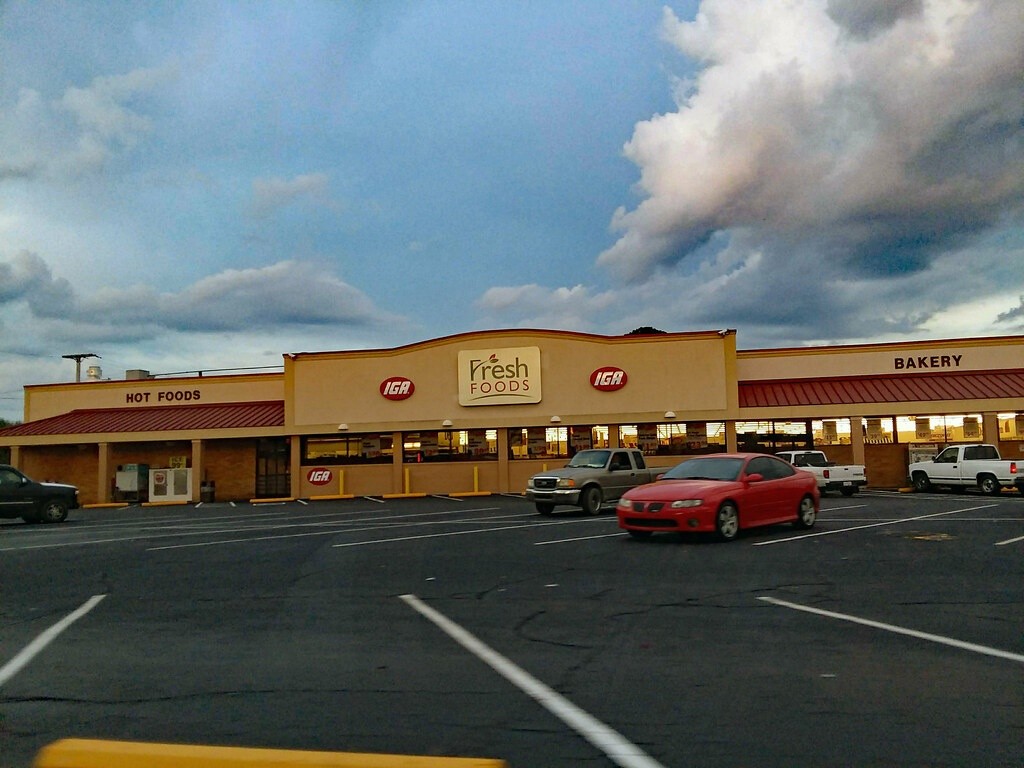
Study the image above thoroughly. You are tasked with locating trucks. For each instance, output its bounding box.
[525,448,675,516]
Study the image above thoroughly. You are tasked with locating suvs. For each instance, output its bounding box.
[0,464,81,523]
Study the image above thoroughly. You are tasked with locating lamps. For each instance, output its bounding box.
[550,416,561,423]
[664,411,677,418]
[338,424,349,430]
[442,419,453,427]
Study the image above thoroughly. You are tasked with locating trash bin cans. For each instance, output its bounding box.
[200,480,215,504]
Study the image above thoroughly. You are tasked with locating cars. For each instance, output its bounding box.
[617,452,822,543]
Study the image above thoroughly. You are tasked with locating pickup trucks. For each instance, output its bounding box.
[909,444,1024,497]
[775,450,867,497]
